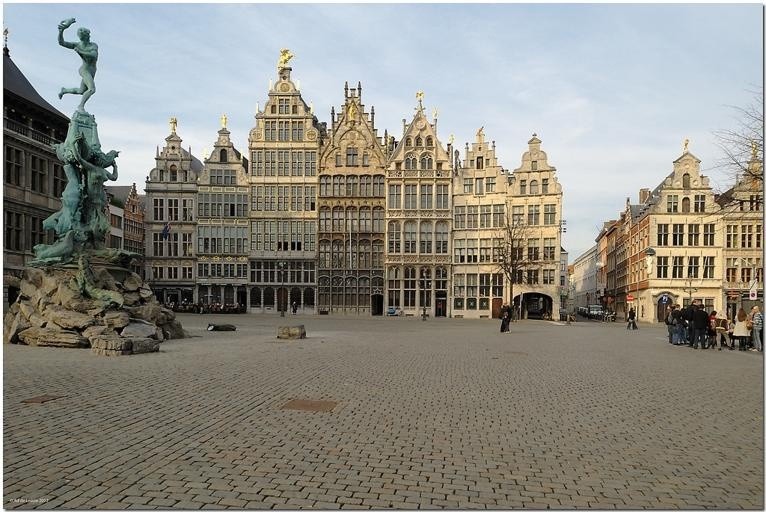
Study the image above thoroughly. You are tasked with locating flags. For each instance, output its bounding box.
[161,223,172,240]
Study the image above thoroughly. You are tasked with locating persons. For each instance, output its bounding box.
[684,138,689,150]
[277,48,293,69]
[416,90,424,99]
[476,126,485,143]
[32,112,118,259]
[219,114,227,128]
[449,133,455,144]
[431,106,439,119]
[747,141,759,158]
[169,117,177,132]
[497,300,763,352]
[348,102,357,120]
[163,291,298,315]
[56,16,98,115]
